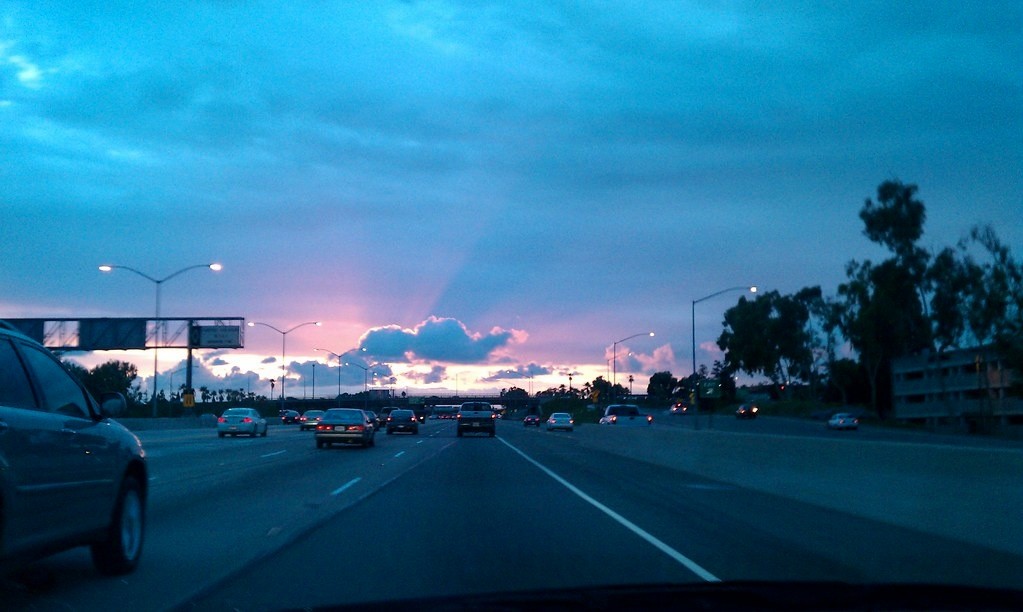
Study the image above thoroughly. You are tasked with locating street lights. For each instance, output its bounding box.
[608,353,631,382]
[507,370,531,396]
[98,262,222,417]
[613,331,655,386]
[691,287,758,430]
[345,362,378,407]
[315,347,367,407]
[247,320,322,414]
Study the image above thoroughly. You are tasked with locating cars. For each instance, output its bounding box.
[599,405,653,428]
[282,411,301,425]
[669,403,688,416]
[0,327,149,576]
[300,410,325,431]
[524,415,540,427]
[414,407,457,425]
[379,407,401,426]
[735,404,759,420]
[216,408,269,437]
[828,413,859,431]
[547,413,574,432]
[385,410,418,435]
[315,408,374,451]
[365,411,380,431]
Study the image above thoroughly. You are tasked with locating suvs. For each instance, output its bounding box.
[456,402,496,438]
